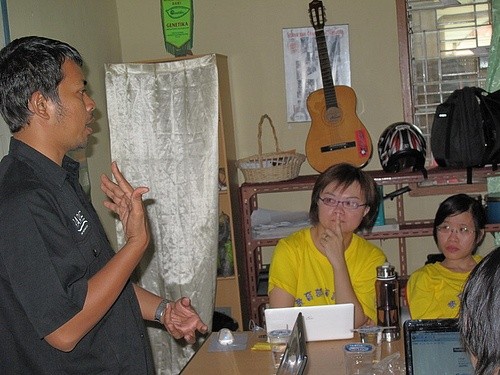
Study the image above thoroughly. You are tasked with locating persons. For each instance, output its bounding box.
[455,245,500,375]
[406,193,485,318]
[0,36,208,375]
[267,163,388,329]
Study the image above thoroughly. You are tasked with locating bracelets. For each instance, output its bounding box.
[154,299,170,322]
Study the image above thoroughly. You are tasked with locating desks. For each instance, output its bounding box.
[179,330,405,375]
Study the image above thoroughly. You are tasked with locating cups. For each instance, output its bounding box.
[268,329,292,369]
[344,343,375,375]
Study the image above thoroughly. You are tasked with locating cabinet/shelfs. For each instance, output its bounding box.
[104,52,245,334]
[242,167,500,330]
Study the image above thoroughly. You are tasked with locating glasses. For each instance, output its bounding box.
[436,225,478,235]
[319,195,369,209]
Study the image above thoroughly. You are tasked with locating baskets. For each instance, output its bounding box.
[234,113,305,183]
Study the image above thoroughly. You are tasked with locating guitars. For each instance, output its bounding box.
[303,1,373,174]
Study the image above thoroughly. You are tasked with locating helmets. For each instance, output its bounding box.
[378,122,428,173]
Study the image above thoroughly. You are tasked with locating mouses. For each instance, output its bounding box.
[216,328,234,345]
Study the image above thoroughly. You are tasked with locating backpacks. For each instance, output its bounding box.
[431,86,500,184]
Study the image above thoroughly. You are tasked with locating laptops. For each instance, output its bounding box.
[264,304,354,375]
[403,317,478,375]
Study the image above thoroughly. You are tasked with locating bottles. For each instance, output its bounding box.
[375,264,401,341]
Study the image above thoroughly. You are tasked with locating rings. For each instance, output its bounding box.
[325,235,329,239]
